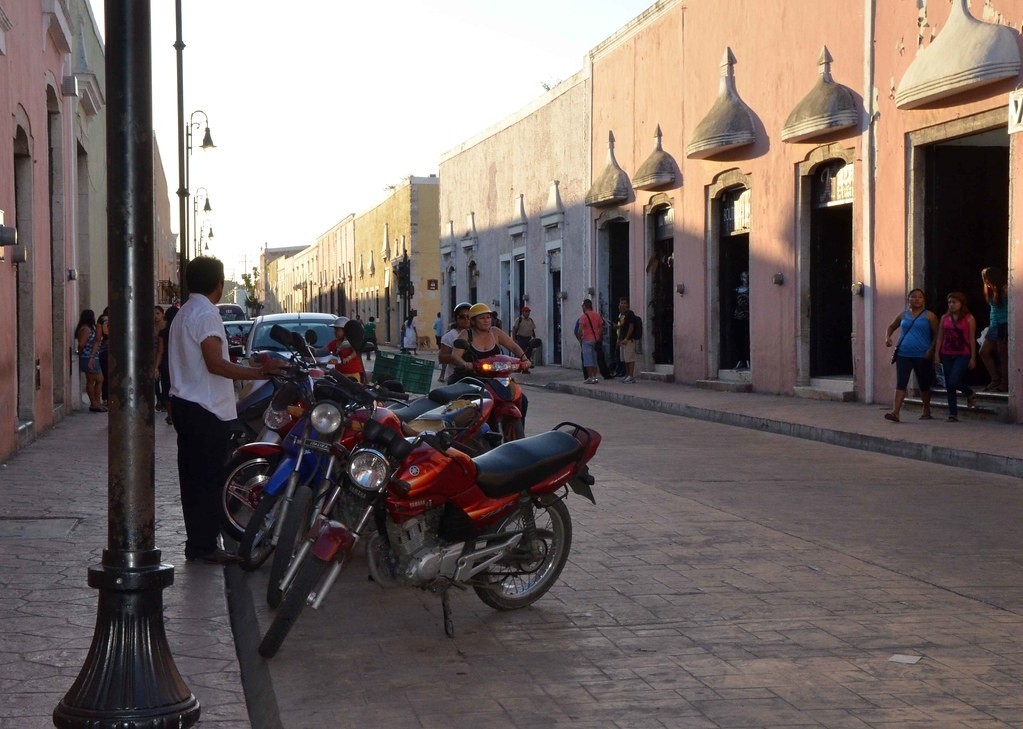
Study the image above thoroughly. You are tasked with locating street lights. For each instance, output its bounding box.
[198,239,209,257]
[194,187,213,258]
[199,219,214,257]
[186,110,217,260]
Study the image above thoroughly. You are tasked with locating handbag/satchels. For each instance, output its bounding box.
[966,351,986,375]
[890,346,899,365]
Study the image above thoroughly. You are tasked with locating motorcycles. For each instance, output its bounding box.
[257,368,602,660]
[220,325,504,612]
[452,338,543,450]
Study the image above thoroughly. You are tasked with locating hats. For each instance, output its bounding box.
[521,307,532,311]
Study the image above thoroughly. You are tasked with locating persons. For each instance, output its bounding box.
[74,310,108,412]
[512,307,536,374]
[578,299,603,384]
[451,303,532,430]
[979,267,1008,393]
[364,317,378,360]
[168,255,289,565]
[154,305,179,425]
[314,317,364,383]
[574,303,615,380]
[151,305,167,412]
[614,304,626,377]
[884,288,938,422]
[433,312,441,350]
[88,306,109,410]
[404,313,419,355]
[438,302,472,386]
[613,297,636,384]
[935,291,976,422]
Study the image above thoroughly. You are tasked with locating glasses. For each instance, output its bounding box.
[457,314,469,320]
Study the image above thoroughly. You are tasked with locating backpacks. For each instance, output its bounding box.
[632,316,643,340]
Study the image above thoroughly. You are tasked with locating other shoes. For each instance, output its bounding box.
[622,375,627,380]
[184,545,241,565]
[437,377,446,382]
[583,377,598,384]
[522,370,530,374]
[622,375,636,384]
[154,403,167,412]
[604,375,615,379]
[982,379,1000,392]
[615,373,624,377]
[89,404,108,412]
[165,416,173,425]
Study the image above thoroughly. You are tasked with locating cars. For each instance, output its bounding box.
[215,303,246,321]
[228,312,341,390]
[223,320,255,336]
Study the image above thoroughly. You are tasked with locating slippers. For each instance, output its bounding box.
[966,392,977,407]
[945,416,959,422]
[919,415,933,420]
[884,413,900,422]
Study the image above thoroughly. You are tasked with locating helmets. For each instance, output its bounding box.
[453,302,472,321]
[328,317,350,328]
[468,302,492,320]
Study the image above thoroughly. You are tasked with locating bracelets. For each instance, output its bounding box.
[90,357,94,358]
